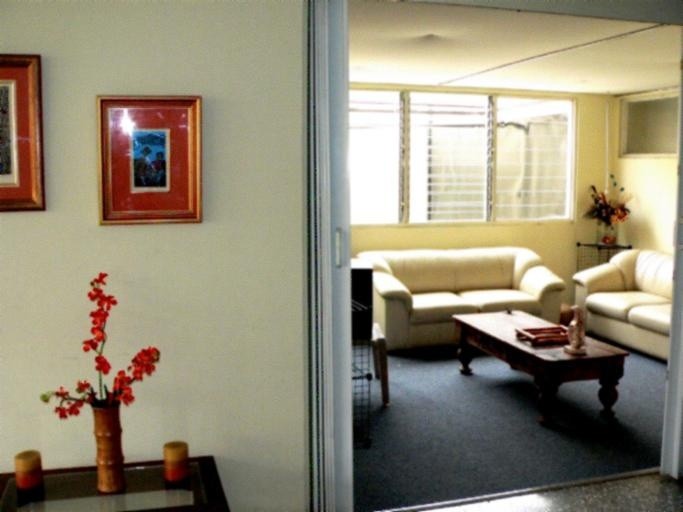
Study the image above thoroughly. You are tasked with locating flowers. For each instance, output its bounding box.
[41,271,160,420]
[583,172,631,231]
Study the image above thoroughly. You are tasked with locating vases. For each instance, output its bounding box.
[602,235,615,243]
[91,399,128,493]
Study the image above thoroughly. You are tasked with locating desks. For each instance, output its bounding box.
[0,455,231,512]
[352,323,390,407]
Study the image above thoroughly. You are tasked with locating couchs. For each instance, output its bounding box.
[352,247,567,351]
[571,248,674,360]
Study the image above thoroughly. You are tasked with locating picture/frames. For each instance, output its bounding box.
[94,96,205,226]
[0,54,46,210]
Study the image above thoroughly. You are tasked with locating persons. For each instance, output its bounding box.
[132,146,157,186]
[148,151,164,186]
